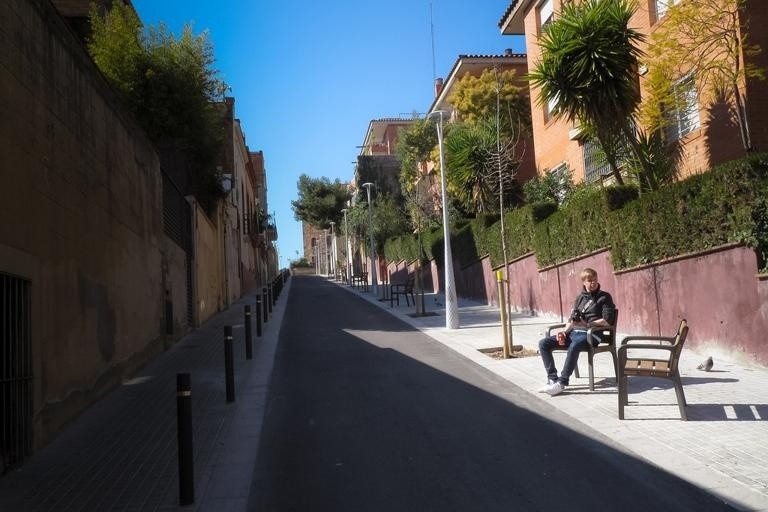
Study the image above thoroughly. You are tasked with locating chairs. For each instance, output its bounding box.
[545,308,690,422]
[350,272,369,292]
[390,275,416,308]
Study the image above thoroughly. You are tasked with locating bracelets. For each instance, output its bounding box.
[586,322,591,329]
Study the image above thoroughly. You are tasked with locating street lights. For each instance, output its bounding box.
[362,182,379,296]
[313,222,338,280]
[429,109,461,329]
[341,209,353,287]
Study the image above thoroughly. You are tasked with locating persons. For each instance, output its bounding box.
[537,266,614,397]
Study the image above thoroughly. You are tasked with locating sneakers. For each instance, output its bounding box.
[545,382,565,395]
[538,379,555,393]
